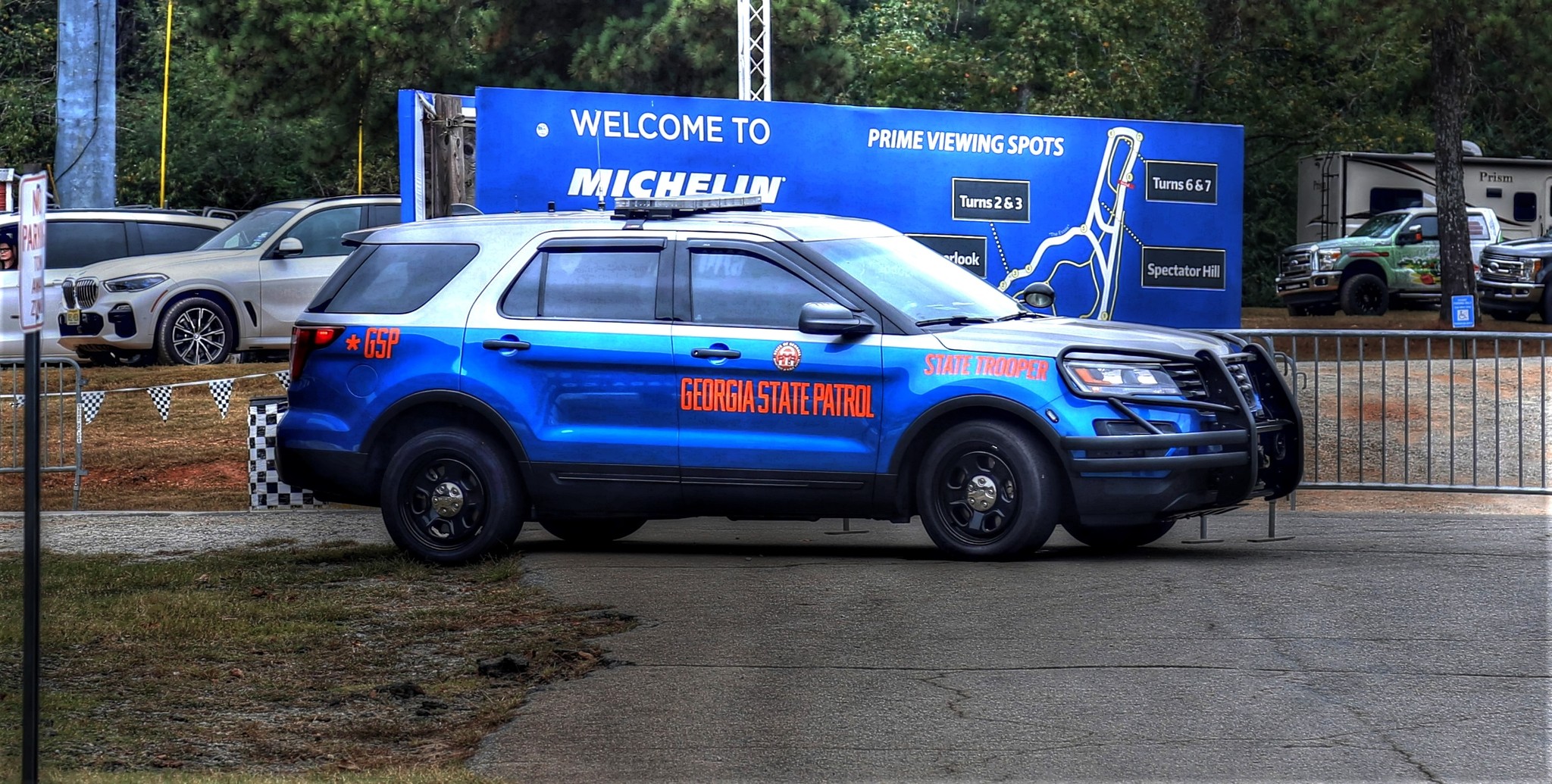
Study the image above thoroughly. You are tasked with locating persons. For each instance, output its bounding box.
[0,236,19,271]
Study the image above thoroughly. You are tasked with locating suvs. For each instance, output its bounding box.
[1273,205,1533,318]
[1478,223,1552,327]
[54,191,404,367]
[271,185,1311,570]
[0,202,258,370]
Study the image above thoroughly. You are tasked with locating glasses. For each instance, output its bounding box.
[0,248,11,252]
